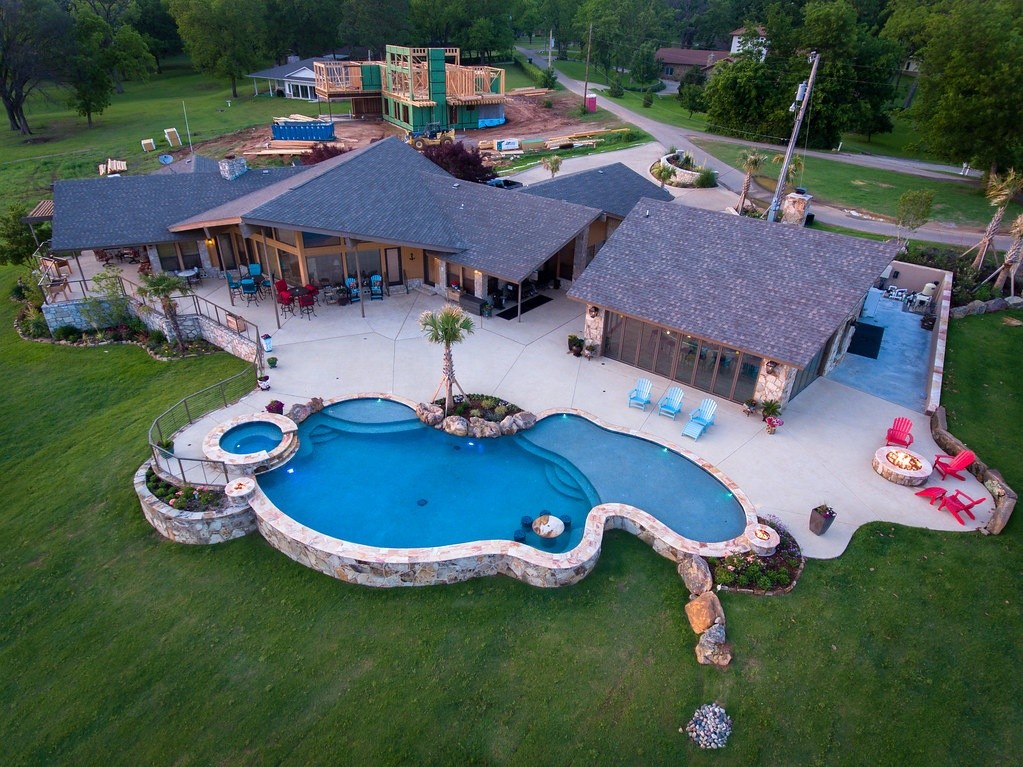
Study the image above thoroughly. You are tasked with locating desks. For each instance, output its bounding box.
[53,258,72,275]
[533,515,565,547]
[115,253,128,262]
[446,287,465,304]
[240,274,264,284]
[177,270,196,292]
[287,287,310,296]
[46,276,72,301]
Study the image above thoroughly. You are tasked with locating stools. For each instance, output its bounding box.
[521,516,530,531]
[559,514,571,528]
[539,510,551,516]
[513,530,526,542]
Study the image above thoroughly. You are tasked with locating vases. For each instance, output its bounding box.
[262,338,273,352]
[765,427,777,434]
[269,410,284,416]
[809,505,837,536]
[451,285,457,288]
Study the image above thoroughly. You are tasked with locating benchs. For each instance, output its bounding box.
[460,295,486,316]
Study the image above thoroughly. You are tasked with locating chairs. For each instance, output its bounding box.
[886,417,914,447]
[92,247,140,266]
[628,377,653,412]
[345,277,360,304]
[175,264,321,320]
[915,484,986,526]
[932,449,975,481]
[658,387,685,420]
[681,399,718,442]
[366,275,384,300]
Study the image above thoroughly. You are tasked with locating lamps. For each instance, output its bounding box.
[588,307,599,319]
[765,361,779,375]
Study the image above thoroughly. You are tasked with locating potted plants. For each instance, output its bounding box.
[572,338,584,356]
[585,345,596,356]
[482,305,493,317]
[567,334,578,351]
[267,357,279,368]
[257,373,271,390]
[762,399,782,422]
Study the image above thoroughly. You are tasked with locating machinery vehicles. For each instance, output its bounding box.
[405,122,455,149]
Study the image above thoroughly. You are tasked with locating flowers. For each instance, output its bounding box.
[765,416,784,427]
[450,280,458,285]
[816,503,837,517]
[265,400,284,411]
[260,333,271,340]
